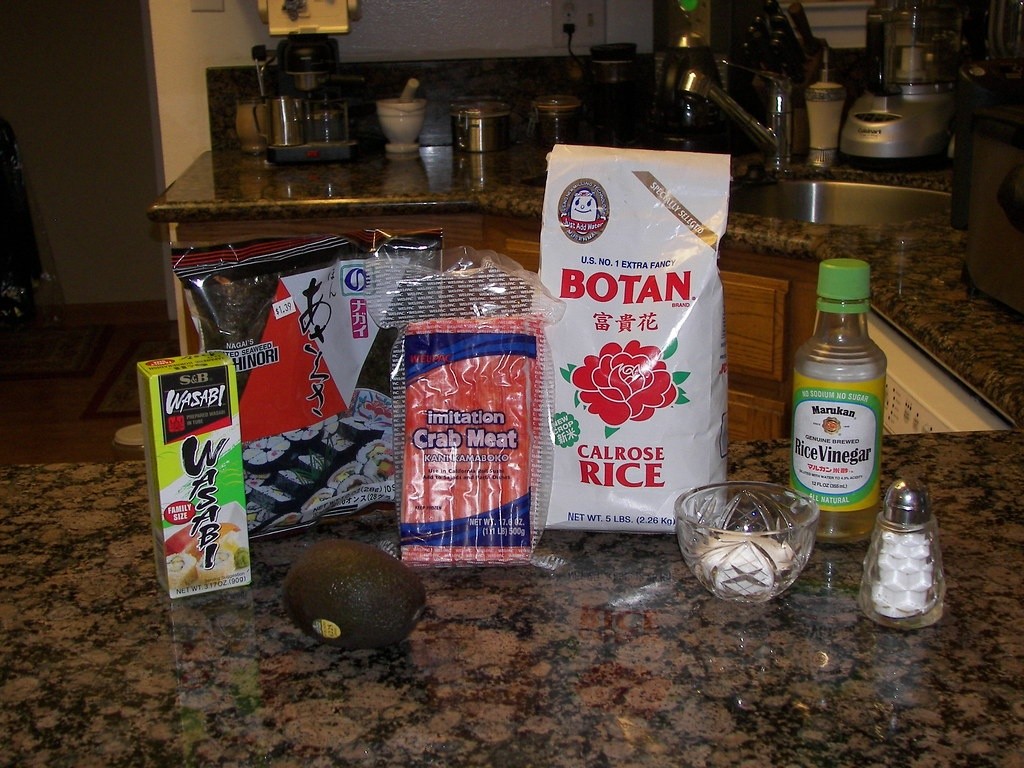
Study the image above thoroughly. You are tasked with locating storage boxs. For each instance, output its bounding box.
[135,353,252,600]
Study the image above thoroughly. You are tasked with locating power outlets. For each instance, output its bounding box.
[553,0,607,49]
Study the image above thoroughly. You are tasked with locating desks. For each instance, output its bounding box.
[1,428,1024,768]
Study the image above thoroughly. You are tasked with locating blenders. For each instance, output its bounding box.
[840,0,956,159]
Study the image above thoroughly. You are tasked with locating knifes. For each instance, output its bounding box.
[743,0,820,83]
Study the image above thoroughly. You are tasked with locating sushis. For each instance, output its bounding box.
[240,404,395,538]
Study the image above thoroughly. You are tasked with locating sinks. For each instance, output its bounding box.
[729,169,951,227]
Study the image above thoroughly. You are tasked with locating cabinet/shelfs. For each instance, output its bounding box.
[172,216,795,449]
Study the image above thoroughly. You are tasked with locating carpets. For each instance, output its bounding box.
[0,323,115,382]
[77,338,181,422]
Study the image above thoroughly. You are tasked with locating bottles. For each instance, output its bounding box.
[788,259,888,546]
[857,475,947,629]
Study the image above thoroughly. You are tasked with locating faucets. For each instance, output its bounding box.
[676,57,794,165]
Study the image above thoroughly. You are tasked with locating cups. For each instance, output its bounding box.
[252,95,305,147]
[236,97,269,153]
[306,101,348,145]
[806,81,844,150]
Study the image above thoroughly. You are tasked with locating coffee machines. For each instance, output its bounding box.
[252,33,354,163]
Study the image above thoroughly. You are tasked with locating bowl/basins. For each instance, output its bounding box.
[674,481,820,605]
[527,110,575,149]
[450,100,514,152]
